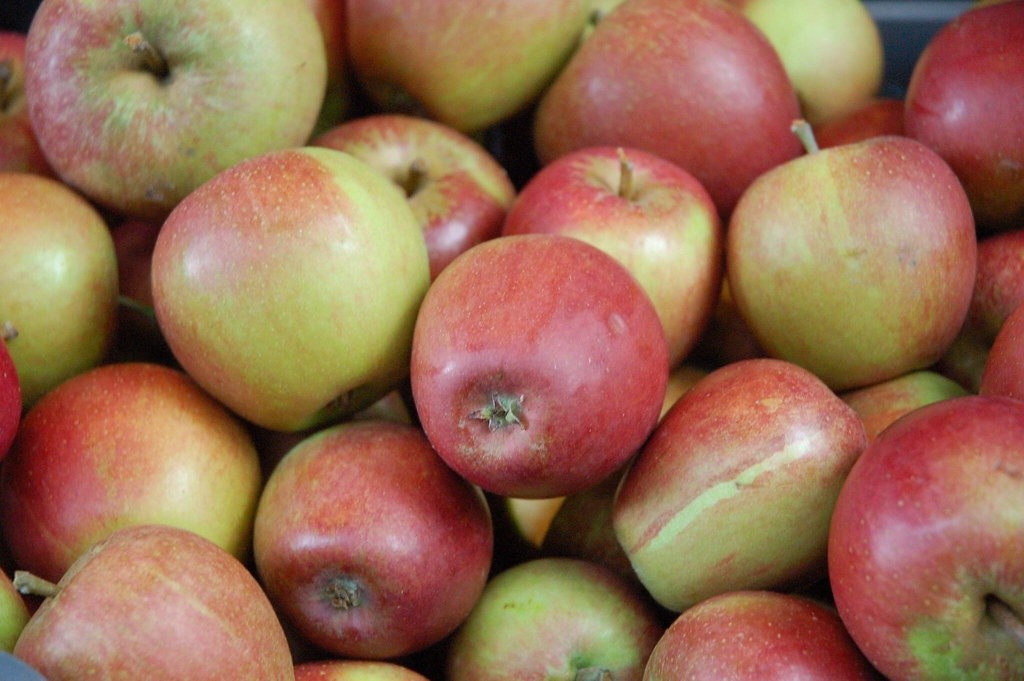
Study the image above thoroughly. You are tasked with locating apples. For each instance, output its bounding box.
[0,0,1024,681]
